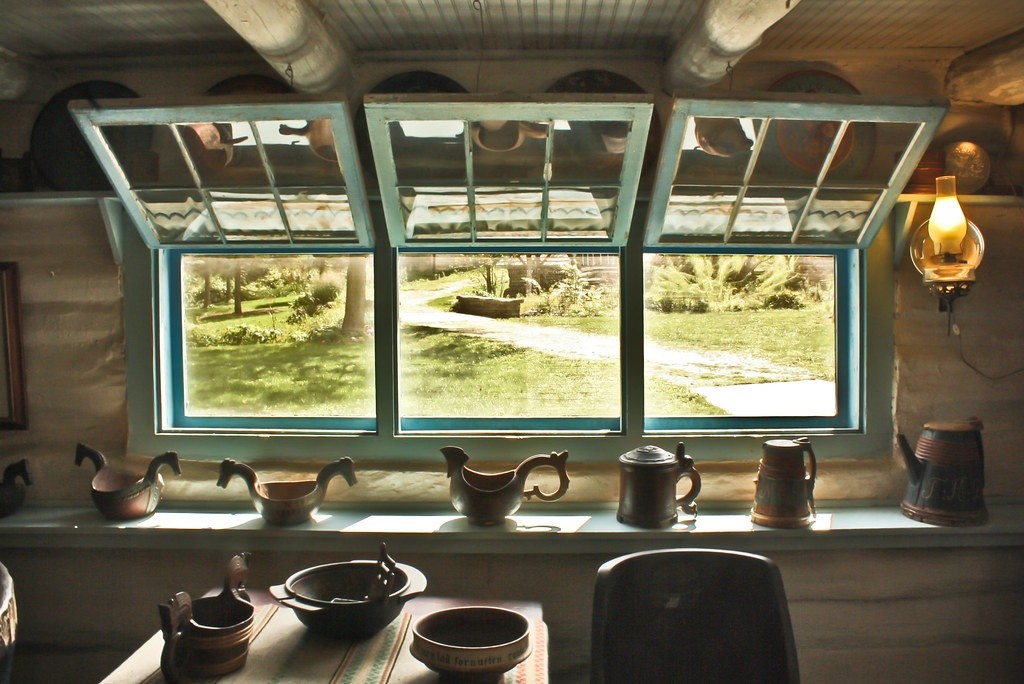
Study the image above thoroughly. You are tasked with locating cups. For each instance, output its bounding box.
[750,437,817,528]
[616,441,701,528]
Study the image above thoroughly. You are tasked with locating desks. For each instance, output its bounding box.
[95,584,552,684]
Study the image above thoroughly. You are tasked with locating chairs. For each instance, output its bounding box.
[588,546,802,684]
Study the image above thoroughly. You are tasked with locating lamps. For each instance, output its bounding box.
[907,173,988,338]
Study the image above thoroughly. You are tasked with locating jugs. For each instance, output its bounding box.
[897,417,990,527]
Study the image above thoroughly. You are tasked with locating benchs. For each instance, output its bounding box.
[455,295,524,319]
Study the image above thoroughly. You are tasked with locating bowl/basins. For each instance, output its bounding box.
[0,458,33,520]
[440,446,570,528]
[269,559,428,639]
[409,606,533,684]
[217,457,358,526]
[74,442,182,520]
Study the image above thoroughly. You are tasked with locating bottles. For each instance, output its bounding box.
[894,150,943,194]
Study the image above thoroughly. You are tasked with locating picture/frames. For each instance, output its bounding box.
[0,261,29,433]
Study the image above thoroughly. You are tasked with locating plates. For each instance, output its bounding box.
[183,75,329,186]
[751,70,876,181]
[31,81,154,191]
[354,71,484,181]
[544,69,662,180]
